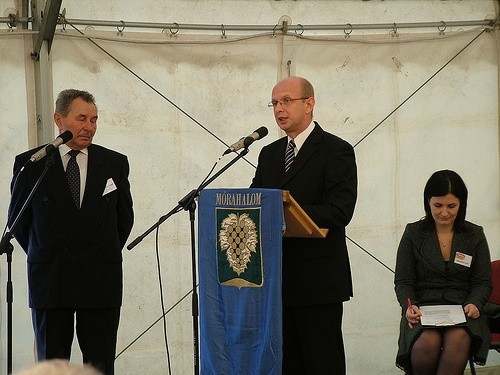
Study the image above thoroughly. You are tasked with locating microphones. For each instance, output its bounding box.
[223,126,268,155]
[25,131,73,166]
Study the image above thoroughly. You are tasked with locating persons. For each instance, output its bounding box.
[247,76,358,375]
[395,169,493,375]
[8,89,134,375]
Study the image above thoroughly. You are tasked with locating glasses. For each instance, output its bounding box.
[268,97,309,109]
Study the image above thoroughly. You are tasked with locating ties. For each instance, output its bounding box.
[65,150,81,210]
[285,140,296,173]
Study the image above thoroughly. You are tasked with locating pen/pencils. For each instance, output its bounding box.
[407,297,415,322]
